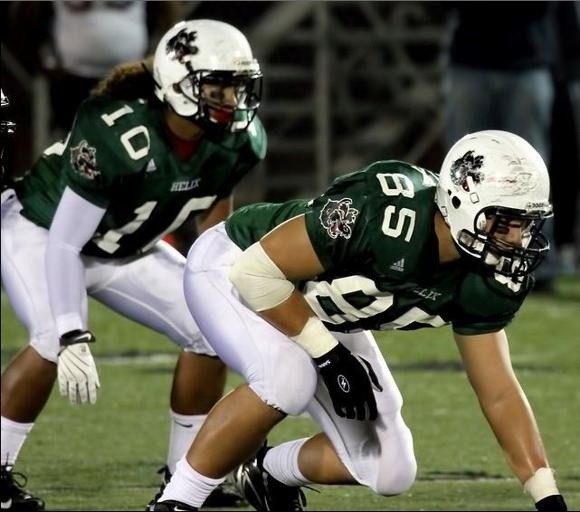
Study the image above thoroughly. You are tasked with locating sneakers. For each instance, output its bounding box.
[144,467,250,511]
[0,461,46,511]
[234,444,307,511]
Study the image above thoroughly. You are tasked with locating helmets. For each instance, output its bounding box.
[436,129,554,277]
[153,17,264,135]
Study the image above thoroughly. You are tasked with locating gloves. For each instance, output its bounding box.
[58,331,101,407]
[314,340,383,421]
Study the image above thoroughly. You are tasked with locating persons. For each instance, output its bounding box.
[422,2,580,299]
[2,2,170,133]
[0,20,269,511]
[146,128,569,512]
